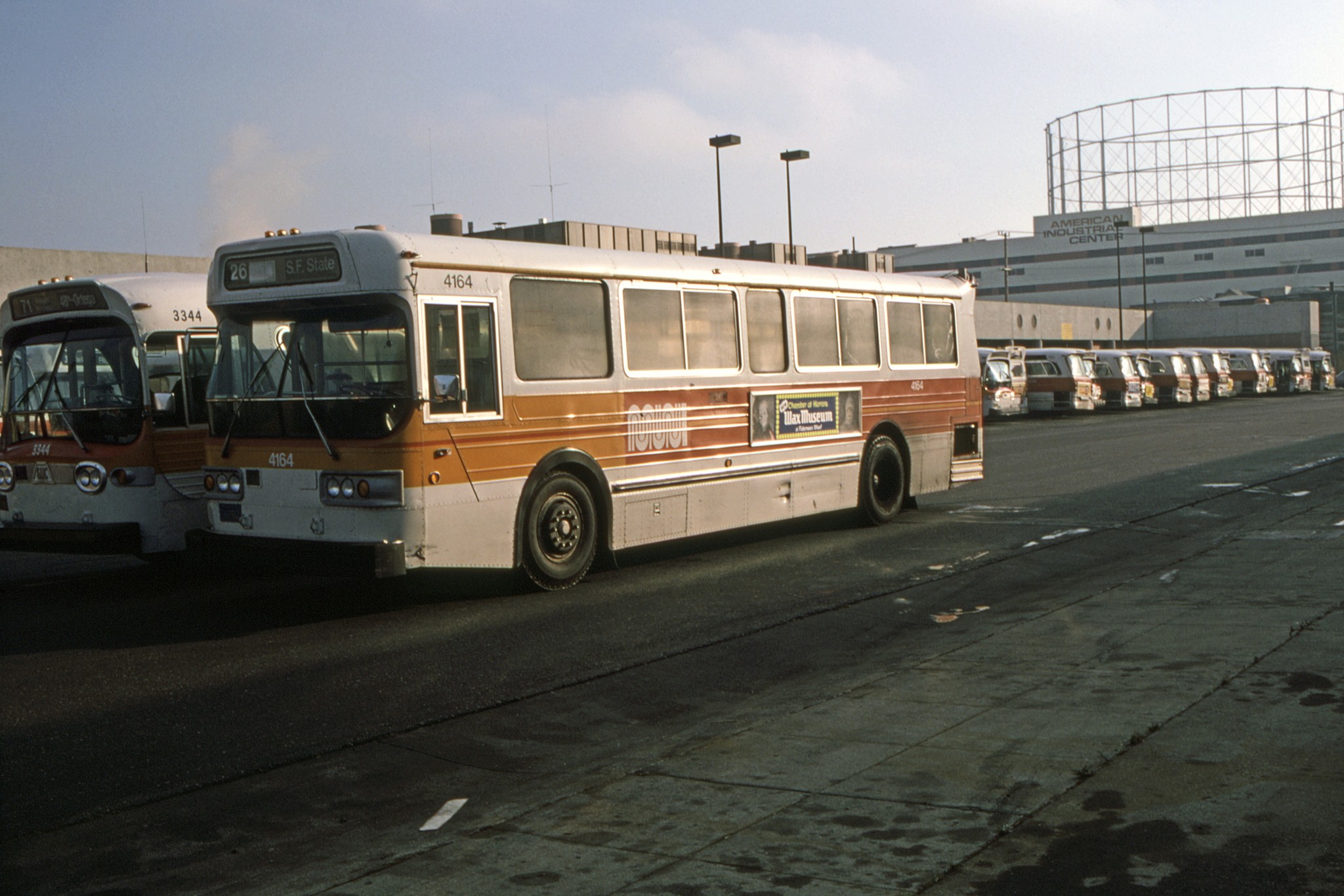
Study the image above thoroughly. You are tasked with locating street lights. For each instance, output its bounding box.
[1139,226,1157,348]
[780,148,811,265]
[1113,220,1129,349]
[709,134,741,260]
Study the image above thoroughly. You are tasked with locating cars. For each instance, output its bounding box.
[1336,371,1344,385]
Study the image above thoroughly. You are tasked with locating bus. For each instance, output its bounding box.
[202,228,984,595]
[0,197,223,549]
[1093,348,1337,409]
[977,346,1022,419]
[1026,347,1106,414]
